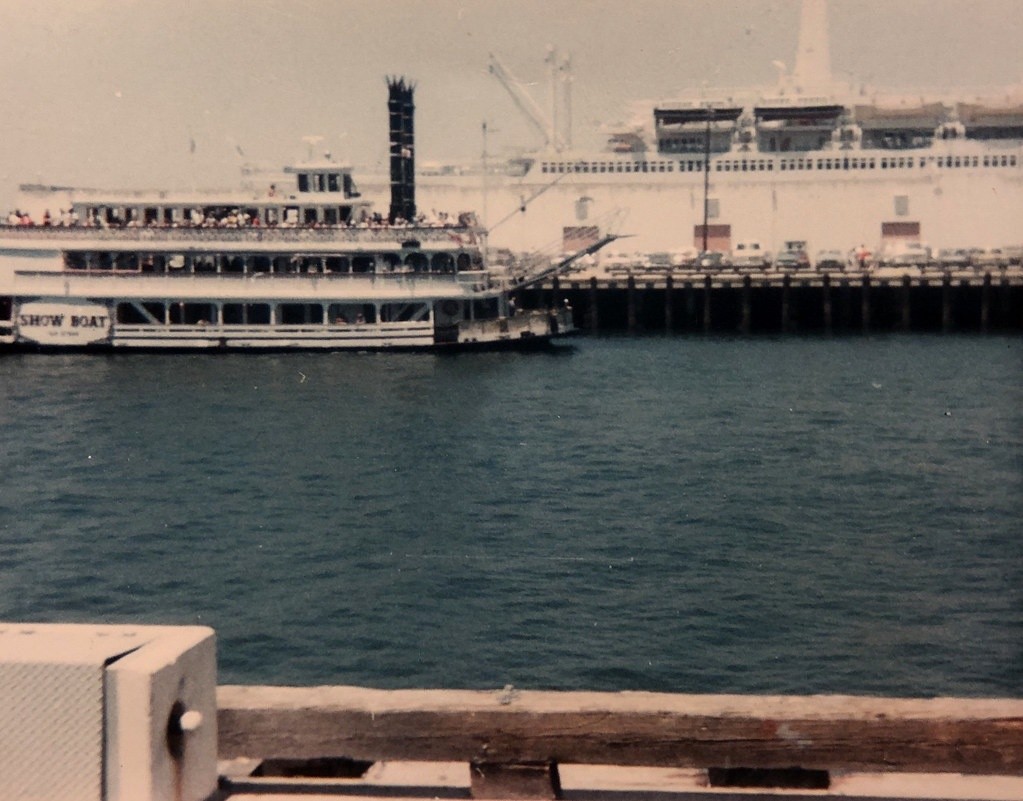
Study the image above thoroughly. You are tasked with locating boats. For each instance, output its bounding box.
[0,149,621,355]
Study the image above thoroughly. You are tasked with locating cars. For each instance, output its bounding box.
[603,252,633,274]
[640,244,1023,274]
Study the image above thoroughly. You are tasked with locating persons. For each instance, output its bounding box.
[354,313,367,325]
[195,316,211,325]
[1,207,475,230]
[90,254,485,273]
[508,296,517,318]
[269,184,278,195]
[335,317,347,326]
